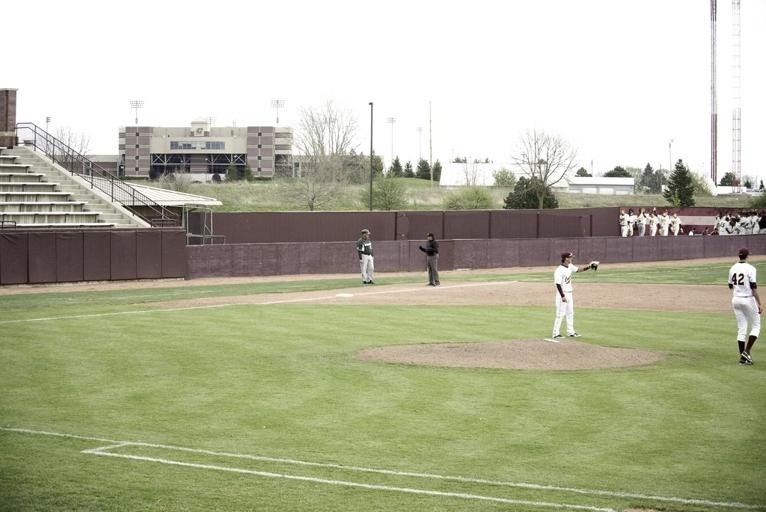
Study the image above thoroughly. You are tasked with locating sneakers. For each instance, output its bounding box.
[739,360,752,365]
[553,335,565,339]
[363,281,374,284]
[741,350,753,363]
[570,333,580,337]
[427,281,440,286]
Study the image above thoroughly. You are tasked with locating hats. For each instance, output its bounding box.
[361,228,371,234]
[426,232,433,236]
[739,248,752,255]
[561,252,574,258]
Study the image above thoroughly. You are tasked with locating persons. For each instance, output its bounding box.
[357,228,374,284]
[420,233,440,286]
[619,208,763,236]
[729,248,763,365]
[552,252,598,338]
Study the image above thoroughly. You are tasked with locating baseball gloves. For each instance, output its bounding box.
[589,261,600,270]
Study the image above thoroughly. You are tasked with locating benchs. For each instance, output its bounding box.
[0,145,113,229]
